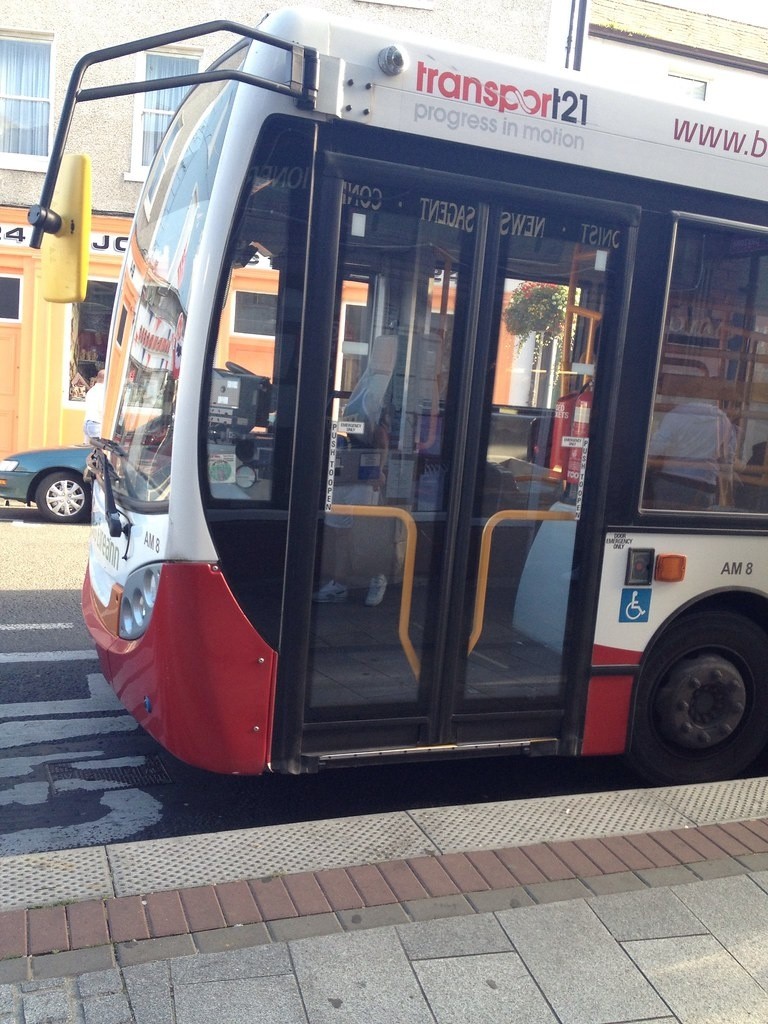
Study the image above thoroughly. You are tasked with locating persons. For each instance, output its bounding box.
[85,370,105,439]
[312,387,391,606]
[648,374,738,510]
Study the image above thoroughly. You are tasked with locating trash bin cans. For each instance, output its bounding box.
[210,368,267,433]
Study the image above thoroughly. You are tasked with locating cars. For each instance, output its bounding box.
[0,446,96,521]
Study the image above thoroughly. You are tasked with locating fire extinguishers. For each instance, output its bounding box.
[565,377,596,483]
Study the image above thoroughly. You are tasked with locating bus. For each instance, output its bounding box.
[28,6,768,786]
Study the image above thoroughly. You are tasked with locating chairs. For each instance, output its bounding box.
[648,455,716,507]
[343,331,441,460]
